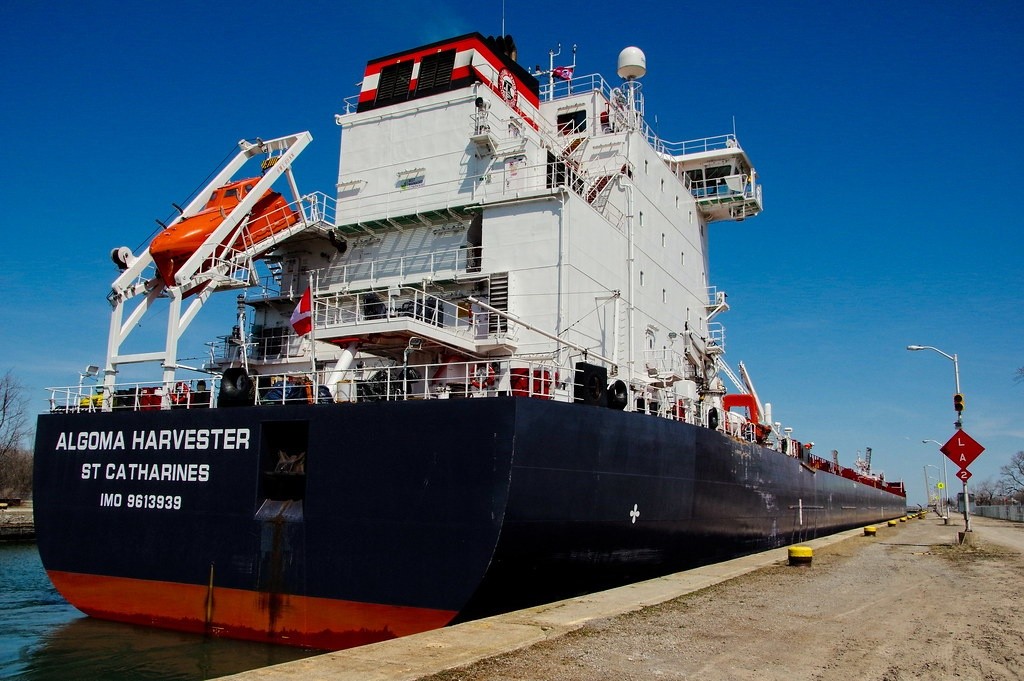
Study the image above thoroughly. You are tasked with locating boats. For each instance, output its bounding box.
[150,176,297,300]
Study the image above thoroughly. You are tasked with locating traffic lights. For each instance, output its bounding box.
[953,393,964,412]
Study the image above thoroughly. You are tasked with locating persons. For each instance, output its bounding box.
[804,442,815,450]
[879,478,886,490]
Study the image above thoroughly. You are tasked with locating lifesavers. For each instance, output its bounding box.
[172,382,189,402]
[470,364,495,388]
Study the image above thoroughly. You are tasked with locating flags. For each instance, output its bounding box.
[290,286,311,337]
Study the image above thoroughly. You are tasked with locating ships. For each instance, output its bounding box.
[32,0,906,650]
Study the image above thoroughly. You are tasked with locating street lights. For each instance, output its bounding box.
[923,440,950,519]
[907,345,971,532]
[924,464,944,516]
